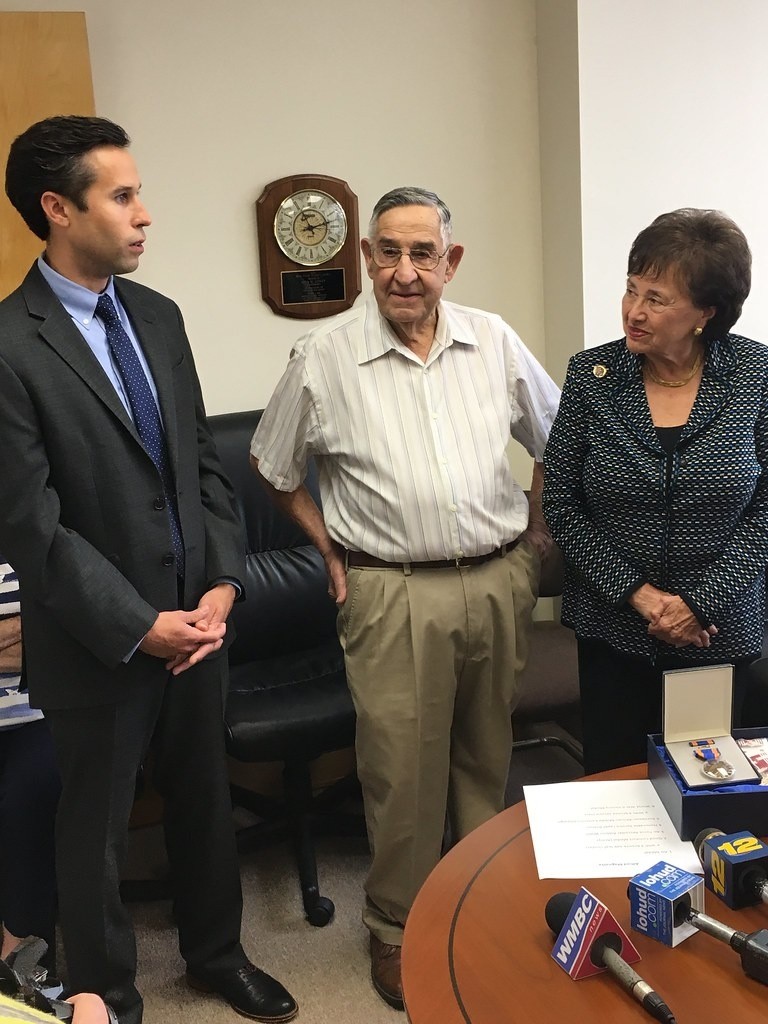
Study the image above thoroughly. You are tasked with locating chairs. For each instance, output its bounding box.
[205,407,362,924]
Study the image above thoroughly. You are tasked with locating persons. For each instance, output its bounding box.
[542,208,768,776]
[0,114,300,1024]
[249,187,562,1009]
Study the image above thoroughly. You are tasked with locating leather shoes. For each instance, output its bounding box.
[185,960,300,1024]
[370,931,405,1011]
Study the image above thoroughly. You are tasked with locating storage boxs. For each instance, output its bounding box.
[647,727,768,842]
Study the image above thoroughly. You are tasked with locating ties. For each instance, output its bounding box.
[94,293,185,582]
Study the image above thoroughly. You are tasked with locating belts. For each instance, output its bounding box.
[348,538,519,569]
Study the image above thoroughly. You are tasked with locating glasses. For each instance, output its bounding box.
[369,244,453,272]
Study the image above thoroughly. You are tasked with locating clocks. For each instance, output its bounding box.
[256,174,362,320]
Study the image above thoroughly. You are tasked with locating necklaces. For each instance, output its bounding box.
[642,346,702,386]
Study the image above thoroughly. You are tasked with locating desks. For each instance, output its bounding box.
[400,762,768,1024]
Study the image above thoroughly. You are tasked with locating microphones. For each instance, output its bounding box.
[544,886,674,1024]
[626,861,768,986]
[691,828,768,911]
